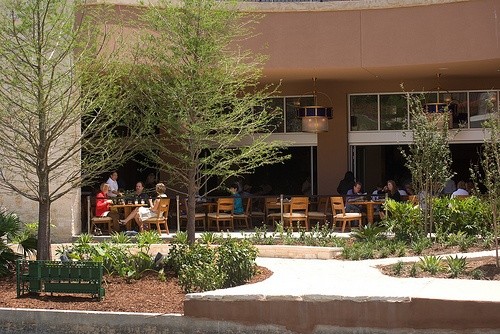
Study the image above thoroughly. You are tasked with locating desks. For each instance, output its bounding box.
[345,200,385,226]
[109,202,149,234]
[266,201,311,229]
[196,201,218,231]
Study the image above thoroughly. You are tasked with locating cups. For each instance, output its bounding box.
[135,199,138,204]
[141,199,145,204]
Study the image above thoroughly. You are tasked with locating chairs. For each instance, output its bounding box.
[253,198,266,228]
[177,200,206,233]
[330,196,363,232]
[141,196,172,236]
[230,198,251,230]
[284,196,310,231]
[207,197,234,232]
[90,205,112,234]
[308,196,329,227]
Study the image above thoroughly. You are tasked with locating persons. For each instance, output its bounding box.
[222,184,243,231]
[106,171,124,200]
[234,177,243,191]
[345,182,367,226]
[118,183,167,232]
[134,182,149,203]
[242,185,250,195]
[372,180,401,225]
[337,171,355,194]
[301,175,311,195]
[450,181,470,199]
[96,183,121,232]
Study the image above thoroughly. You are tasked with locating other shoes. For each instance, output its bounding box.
[117,218,126,226]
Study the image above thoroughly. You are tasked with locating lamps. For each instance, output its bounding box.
[420,71,454,129]
[295,77,334,133]
